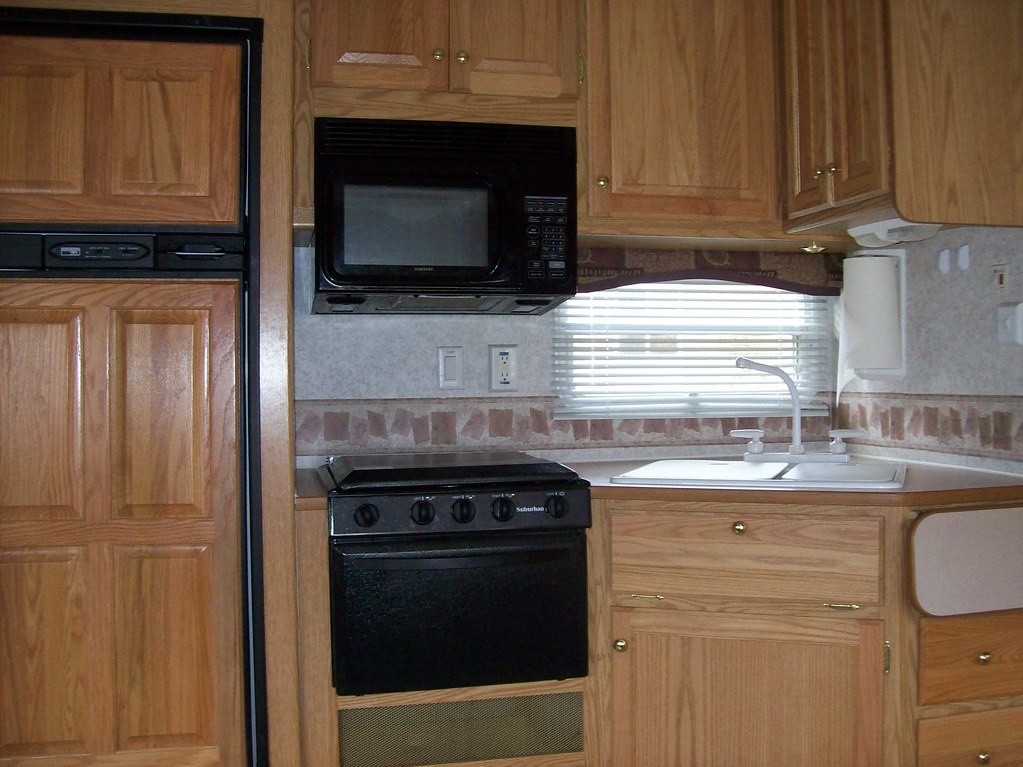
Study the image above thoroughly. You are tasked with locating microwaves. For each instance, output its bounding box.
[310,116,579,315]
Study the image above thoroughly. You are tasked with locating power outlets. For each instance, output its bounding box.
[488,344,518,392]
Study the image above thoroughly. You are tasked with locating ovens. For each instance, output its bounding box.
[316,448,589,695]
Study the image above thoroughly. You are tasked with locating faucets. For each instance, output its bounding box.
[736,358,805,455]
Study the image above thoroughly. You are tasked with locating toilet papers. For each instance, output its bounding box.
[833,252,901,410]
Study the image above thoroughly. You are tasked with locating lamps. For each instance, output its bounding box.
[841,248,908,384]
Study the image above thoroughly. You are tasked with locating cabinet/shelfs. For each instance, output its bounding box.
[593,484,1023,767]
[287,0,1023,254]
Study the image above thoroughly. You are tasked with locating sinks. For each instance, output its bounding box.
[610,458,789,484]
[781,462,907,490]
